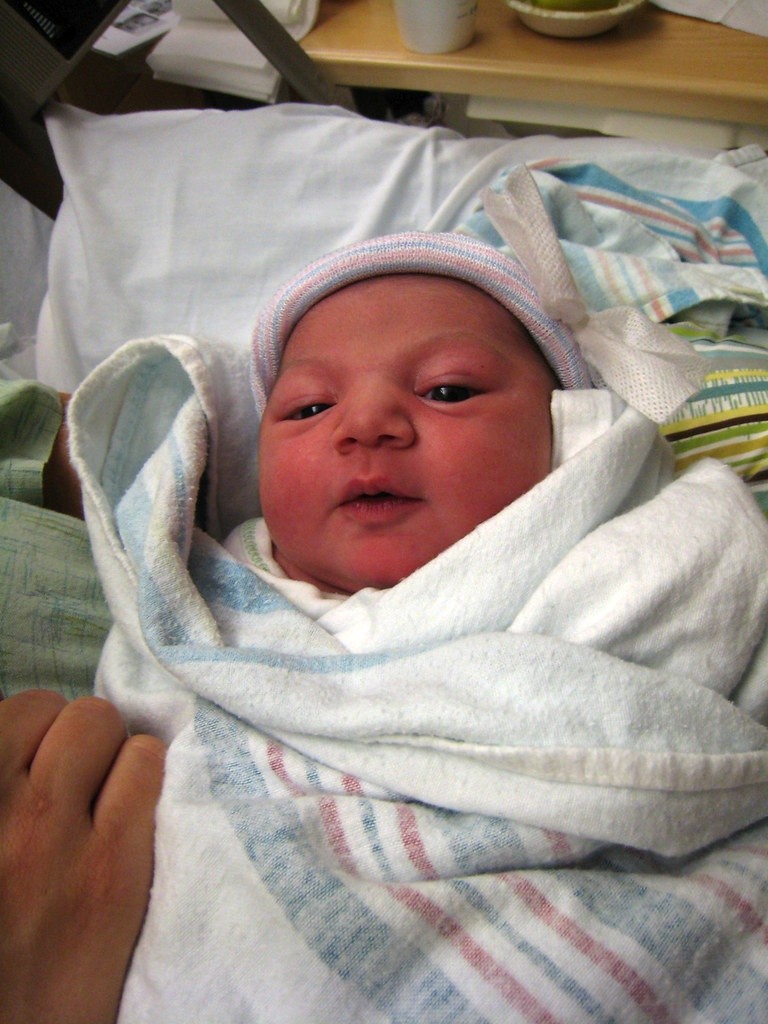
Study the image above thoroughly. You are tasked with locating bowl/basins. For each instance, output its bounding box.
[505,0,642,38]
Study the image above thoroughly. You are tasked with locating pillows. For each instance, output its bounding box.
[34,102,767,478]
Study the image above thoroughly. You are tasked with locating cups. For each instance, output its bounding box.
[394,0,477,54]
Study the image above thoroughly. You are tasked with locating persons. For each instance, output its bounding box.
[65,227,767,1024]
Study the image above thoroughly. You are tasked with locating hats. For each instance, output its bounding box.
[251,165,707,431]
